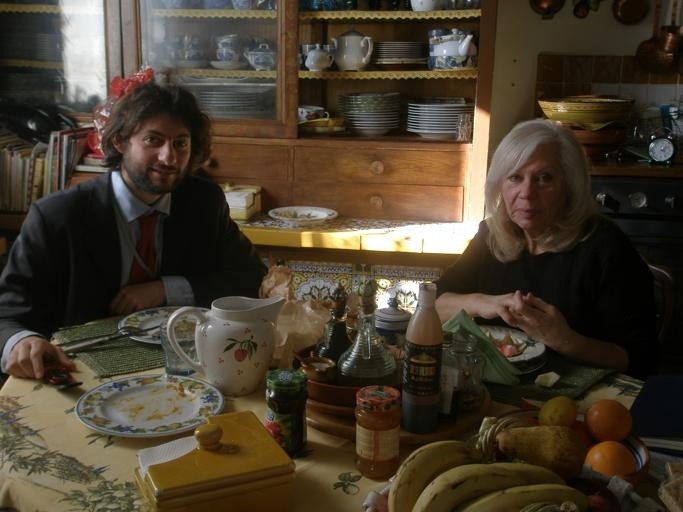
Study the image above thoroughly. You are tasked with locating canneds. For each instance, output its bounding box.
[354,384,401,478]
[264,369,308,456]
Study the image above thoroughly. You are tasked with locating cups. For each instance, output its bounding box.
[374,297,411,337]
[298,104,330,121]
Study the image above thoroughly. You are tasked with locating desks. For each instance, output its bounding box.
[0,292,683,511]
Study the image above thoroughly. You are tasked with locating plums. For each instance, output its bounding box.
[587,495,610,510]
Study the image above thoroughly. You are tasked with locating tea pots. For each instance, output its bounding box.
[329,31,376,73]
[167,293,288,397]
[429,28,478,71]
[303,43,334,72]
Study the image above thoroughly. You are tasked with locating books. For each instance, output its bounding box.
[0,124,112,214]
[628,372,683,457]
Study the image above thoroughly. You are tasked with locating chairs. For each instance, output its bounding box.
[647,263,678,343]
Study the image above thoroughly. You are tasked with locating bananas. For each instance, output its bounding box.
[386,440,587,512]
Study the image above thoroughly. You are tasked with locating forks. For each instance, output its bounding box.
[58,326,150,354]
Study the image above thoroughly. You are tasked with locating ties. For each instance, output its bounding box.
[126,210,159,284]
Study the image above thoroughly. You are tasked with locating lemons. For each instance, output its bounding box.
[538,396,576,427]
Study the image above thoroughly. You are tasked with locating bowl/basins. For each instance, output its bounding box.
[537,95,636,129]
[215,32,242,61]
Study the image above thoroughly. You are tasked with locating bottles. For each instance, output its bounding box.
[336,306,398,379]
[316,281,352,358]
[401,282,444,433]
[443,331,486,419]
[298,355,336,384]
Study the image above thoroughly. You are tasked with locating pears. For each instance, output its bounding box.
[493,425,577,468]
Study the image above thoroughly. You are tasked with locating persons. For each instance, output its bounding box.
[432,117,662,375]
[0,83,269,380]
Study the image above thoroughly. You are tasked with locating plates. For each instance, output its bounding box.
[34,32,64,63]
[268,205,340,227]
[199,91,264,113]
[374,40,426,70]
[498,408,652,488]
[75,372,224,441]
[406,97,475,143]
[335,90,401,135]
[175,57,247,72]
[477,322,547,364]
[118,304,211,346]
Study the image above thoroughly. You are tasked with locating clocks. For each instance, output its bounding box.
[646,126,673,166]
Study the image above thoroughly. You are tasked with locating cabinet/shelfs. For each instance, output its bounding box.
[115,0,496,225]
[0,0,123,231]
